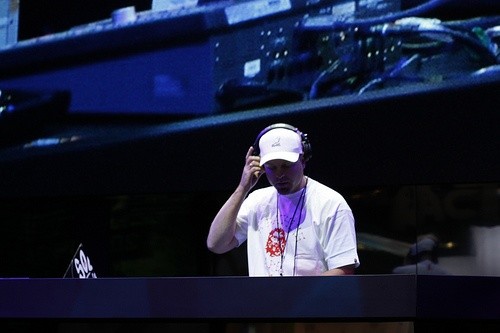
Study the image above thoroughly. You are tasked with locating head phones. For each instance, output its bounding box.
[252,123,313,162]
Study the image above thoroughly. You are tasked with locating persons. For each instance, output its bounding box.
[207,123,361,275]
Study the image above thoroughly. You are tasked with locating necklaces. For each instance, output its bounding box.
[275,178,309,276]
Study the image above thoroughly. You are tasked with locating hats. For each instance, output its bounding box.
[259,123,304,167]
